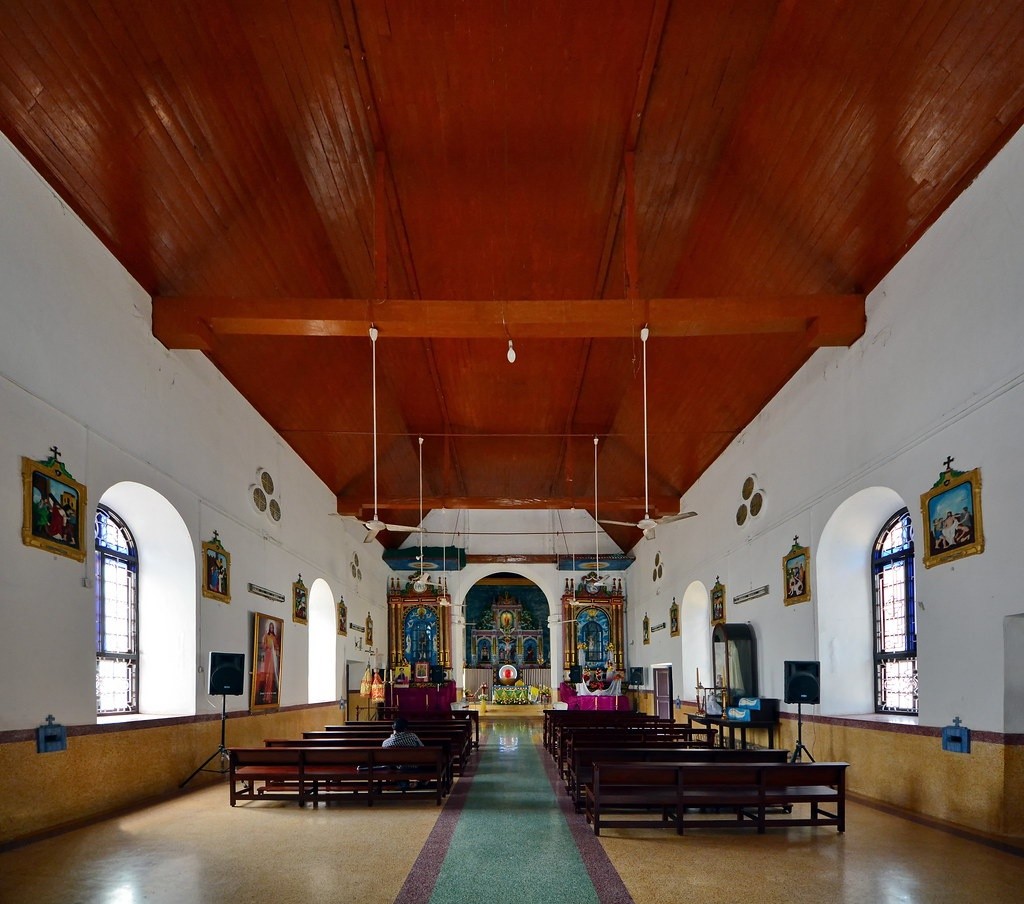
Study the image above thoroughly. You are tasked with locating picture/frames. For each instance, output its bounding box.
[20,456,374,646]
[643,468,985,643]
[249,613,285,714]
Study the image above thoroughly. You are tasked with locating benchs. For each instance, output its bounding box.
[226,720,472,807]
[539,709,850,837]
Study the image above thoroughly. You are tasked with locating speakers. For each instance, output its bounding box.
[783,660,821,705]
[570,665,583,683]
[630,667,645,685]
[208,651,245,696]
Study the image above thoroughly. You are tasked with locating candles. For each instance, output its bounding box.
[616,695,618,706]
[397,695,399,705]
[426,694,428,706]
[697,667,699,687]
[437,683,439,692]
[595,696,597,705]
[390,669,392,682]
[722,666,725,687]
[384,669,386,682]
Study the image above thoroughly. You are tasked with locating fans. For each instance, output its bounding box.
[323,323,697,609]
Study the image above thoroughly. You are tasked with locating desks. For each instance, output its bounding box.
[569,695,629,711]
[685,713,780,750]
[386,680,456,719]
[492,685,530,704]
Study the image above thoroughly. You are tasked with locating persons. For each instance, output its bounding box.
[394,668,405,685]
[382,719,430,790]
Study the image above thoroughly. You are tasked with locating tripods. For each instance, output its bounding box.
[180,695,247,791]
[790,702,834,790]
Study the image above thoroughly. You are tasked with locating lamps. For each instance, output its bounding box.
[651,623,665,632]
[249,584,285,602]
[733,585,769,603]
[350,623,364,632]
[500,290,516,363]
